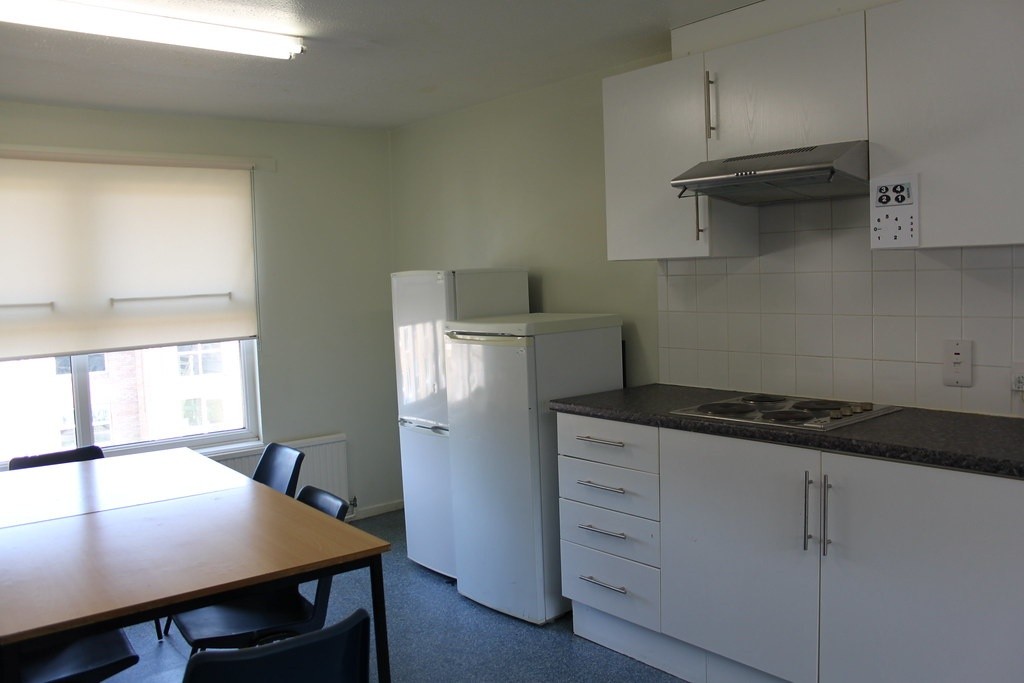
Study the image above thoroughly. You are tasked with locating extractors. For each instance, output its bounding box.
[671,140,868,205]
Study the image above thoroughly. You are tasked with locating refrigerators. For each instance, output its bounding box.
[391,271,531,579]
[444,312,624,625]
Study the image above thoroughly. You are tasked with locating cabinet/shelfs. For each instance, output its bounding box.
[865,0,1024,251]
[556,410,1024,683]
[601,52,761,262]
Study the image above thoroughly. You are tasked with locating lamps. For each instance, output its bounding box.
[0,0,308,61]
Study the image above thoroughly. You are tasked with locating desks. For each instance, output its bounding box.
[0,445,393,683]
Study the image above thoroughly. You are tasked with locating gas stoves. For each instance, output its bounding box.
[673,394,903,431]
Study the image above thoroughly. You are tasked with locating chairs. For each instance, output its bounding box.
[0,628,140,683]
[169,485,351,656]
[162,442,306,652]
[182,608,370,683]
[8,446,163,643]
